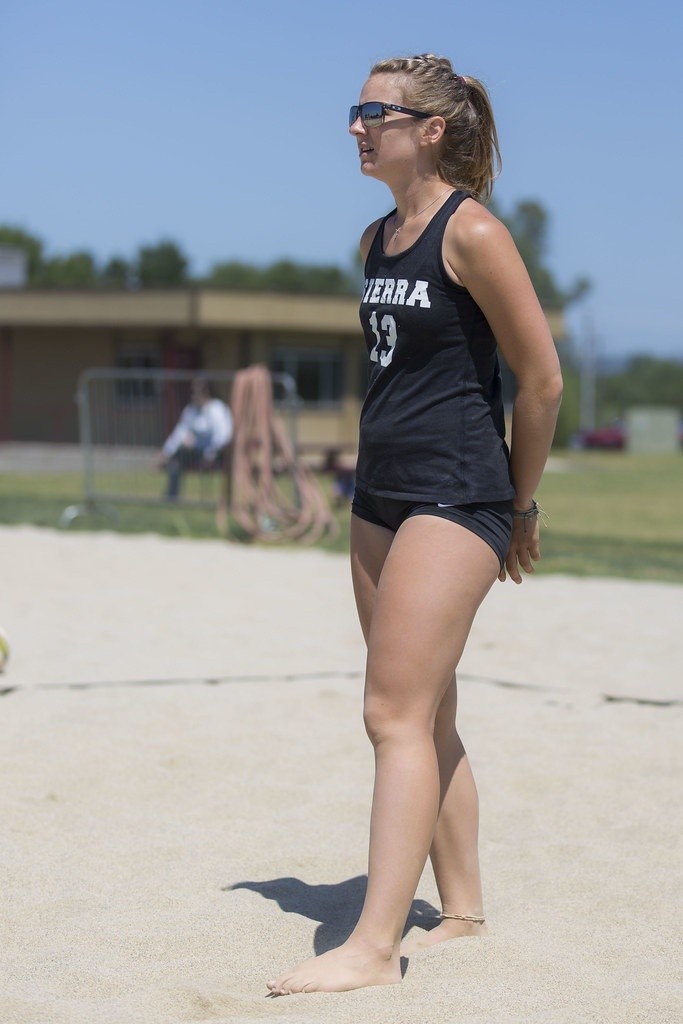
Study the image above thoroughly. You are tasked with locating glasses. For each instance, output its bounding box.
[349,99,448,134]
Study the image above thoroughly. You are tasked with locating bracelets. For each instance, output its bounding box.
[512,499,540,521]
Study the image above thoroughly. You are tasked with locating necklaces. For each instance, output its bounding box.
[393,185,454,243]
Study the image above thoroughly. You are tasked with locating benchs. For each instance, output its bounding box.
[189,455,358,504]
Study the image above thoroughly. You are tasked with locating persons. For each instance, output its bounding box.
[155,377,235,508]
[313,438,367,511]
[265,55,564,1003]
